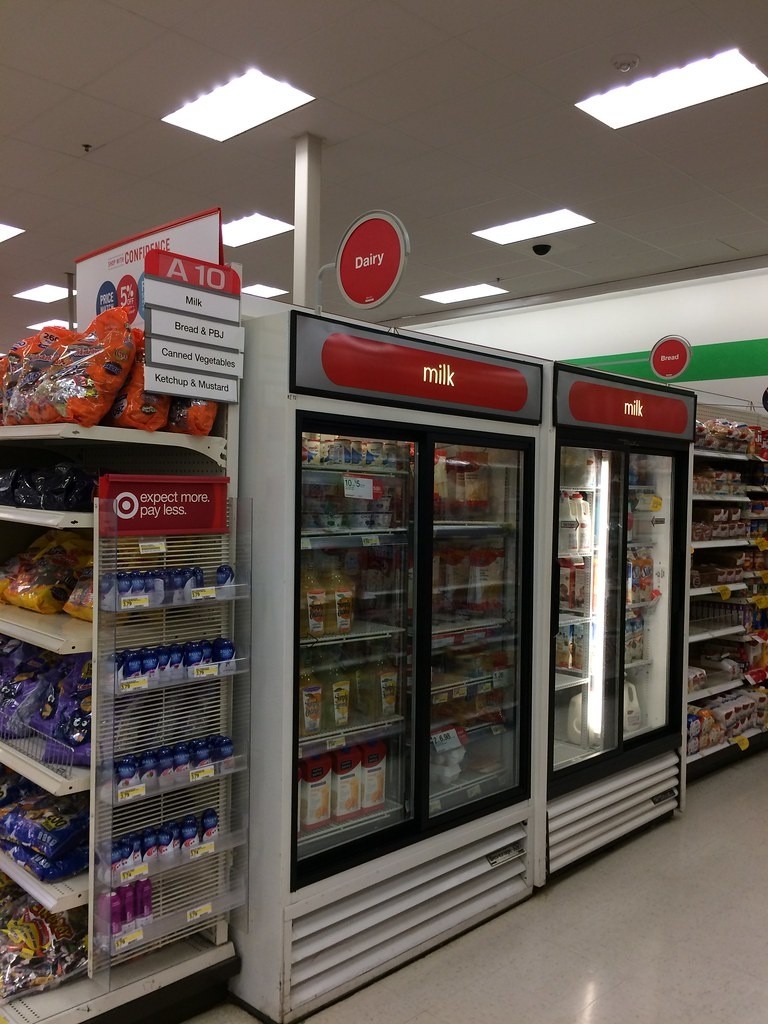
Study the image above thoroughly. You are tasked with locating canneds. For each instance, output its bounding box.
[302,436,397,469]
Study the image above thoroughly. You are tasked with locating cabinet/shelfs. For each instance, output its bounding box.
[686,449,768,765]
[0,422,242,1024]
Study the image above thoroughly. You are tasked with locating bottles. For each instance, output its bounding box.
[623,672,641,732]
[301,551,356,637]
[559,491,591,555]
[299,641,398,737]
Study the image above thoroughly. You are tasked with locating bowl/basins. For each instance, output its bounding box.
[301,492,393,529]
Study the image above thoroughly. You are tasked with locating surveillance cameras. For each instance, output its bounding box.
[533,245,551,255]
